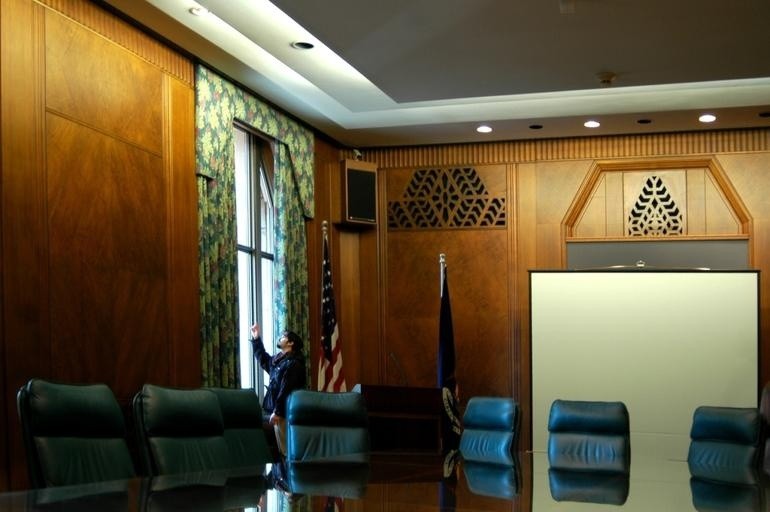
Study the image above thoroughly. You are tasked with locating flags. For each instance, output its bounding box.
[440,452,460,511]
[315,235,349,392]
[322,496,346,511]
[436,267,463,450]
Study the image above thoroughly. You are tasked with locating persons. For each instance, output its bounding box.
[256,462,304,508]
[247,322,305,460]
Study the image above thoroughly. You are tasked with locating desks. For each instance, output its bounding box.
[0,451,770,512]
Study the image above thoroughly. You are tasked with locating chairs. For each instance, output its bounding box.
[284,389,372,467]
[133,383,232,476]
[201,386,271,469]
[686,401,765,466]
[16,378,138,486]
[549,399,632,471]
[459,395,523,468]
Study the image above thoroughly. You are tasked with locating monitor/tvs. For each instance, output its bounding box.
[340,159,378,226]
